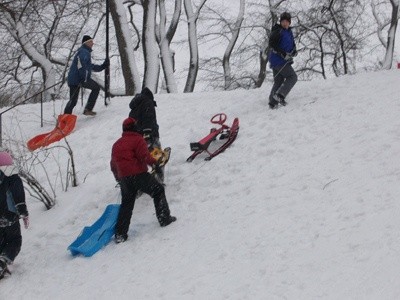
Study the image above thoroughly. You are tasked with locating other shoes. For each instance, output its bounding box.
[84,109,96,115]
[160,216,176,226]
[268,94,287,109]
[115,233,128,242]
[0,259,7,279]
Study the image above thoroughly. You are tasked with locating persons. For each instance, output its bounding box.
[266,11,299,109]
[63,35,110,115]
[128,86,165,199]
[110,117,176,244]
[0,147,30,280]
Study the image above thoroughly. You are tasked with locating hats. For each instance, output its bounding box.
[82,35,93,45]
[0,152,13,166]
[280,12,291,25]
[141,87,154,100]
[123,117,143,131]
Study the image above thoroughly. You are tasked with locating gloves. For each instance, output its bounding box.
[24,216,29,228]
[101,62,109,69]
[284,50,298,61]
[150,128,159,142]
[154,157,163,166]
